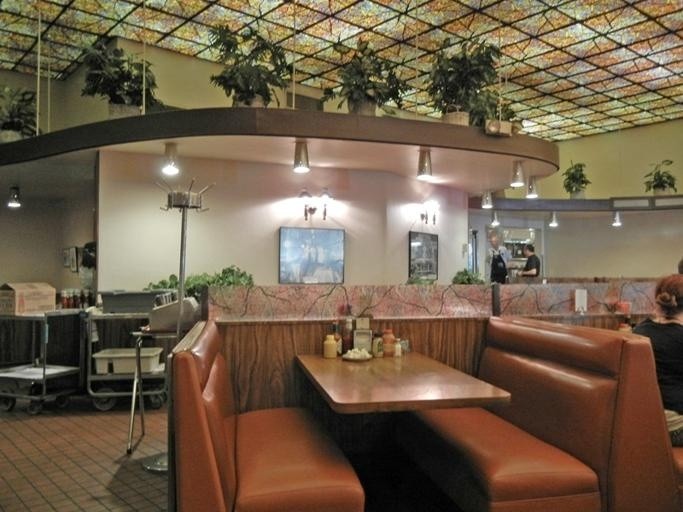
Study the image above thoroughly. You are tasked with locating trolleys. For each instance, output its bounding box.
[85,310,171,411]
[0,308,87,418]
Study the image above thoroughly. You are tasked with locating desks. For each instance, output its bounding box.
[296,346,511,510]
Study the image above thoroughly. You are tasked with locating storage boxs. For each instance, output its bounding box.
[92,346,164,375]
[0,280,57,317]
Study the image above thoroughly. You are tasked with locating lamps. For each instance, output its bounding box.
[475,159,623,233]
[3,185,22,209]
[293,142,311,174]
[160,141,179,177]
[414,149,433,180]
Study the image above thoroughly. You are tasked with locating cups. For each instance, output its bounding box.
[509,269,517,280]
[354,330,372,352]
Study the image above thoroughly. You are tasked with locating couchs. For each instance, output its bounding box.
[171,320,365,509]
[389,308,682,511]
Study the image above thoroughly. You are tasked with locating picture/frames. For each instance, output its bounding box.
[61,247,69,268]
[68,246,78,273]
[406,228,439,283]
[276,225,344,286]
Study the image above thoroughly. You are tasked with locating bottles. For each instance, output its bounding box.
[60,288,91,308]
[323,318,352,358]
[94,293,104,314]
[372,329,401,359]
[392,359,400,372]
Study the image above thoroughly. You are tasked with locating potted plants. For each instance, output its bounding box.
[641,158,678,198]
[77,33,170,119]
[319,37,412,116]
[207,24,295,107]
[0,83,44,147]
[421,19,527,142]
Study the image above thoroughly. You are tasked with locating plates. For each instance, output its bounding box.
[341,353,373,362]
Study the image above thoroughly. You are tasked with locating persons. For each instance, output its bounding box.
[486,236,512,284]
[515,244,540,278]
[628,272,682,449]
